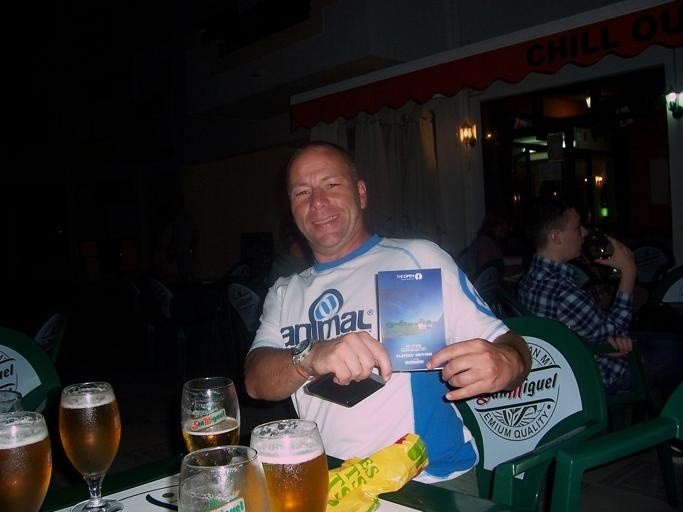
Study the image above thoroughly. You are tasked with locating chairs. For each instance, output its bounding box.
[549,381,682,511]
[476,320,607,509]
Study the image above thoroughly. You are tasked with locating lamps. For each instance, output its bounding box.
[664,86,682,122]
[460,120,476,150]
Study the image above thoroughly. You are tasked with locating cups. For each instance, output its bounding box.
[180,376,242,468]
[0,410,53,511]
[249,418,330,512]
[178,444,272,511]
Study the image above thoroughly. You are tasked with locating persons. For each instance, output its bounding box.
[514,198,683,393]
[242,140,532,496]
[466,211,510,278]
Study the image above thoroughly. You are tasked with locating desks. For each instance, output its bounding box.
[26,452,536,512]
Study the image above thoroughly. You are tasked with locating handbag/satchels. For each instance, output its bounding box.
[326,434,428,512]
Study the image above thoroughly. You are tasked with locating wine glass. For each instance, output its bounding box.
[57,381,125,511]
[586,231,623,279]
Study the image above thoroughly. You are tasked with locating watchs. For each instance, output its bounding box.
[290,337,313,382]
